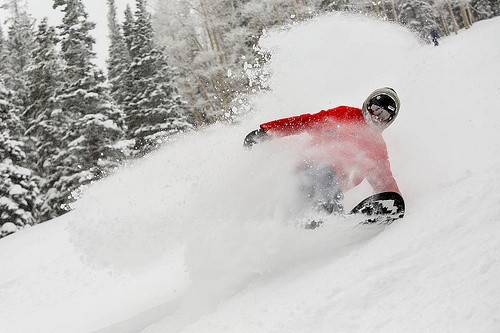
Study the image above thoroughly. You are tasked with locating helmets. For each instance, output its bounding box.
[362,87,400,129]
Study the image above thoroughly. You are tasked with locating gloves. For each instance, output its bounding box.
[243,129,259,147]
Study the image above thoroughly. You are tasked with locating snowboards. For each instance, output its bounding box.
[305,192,405,231]
